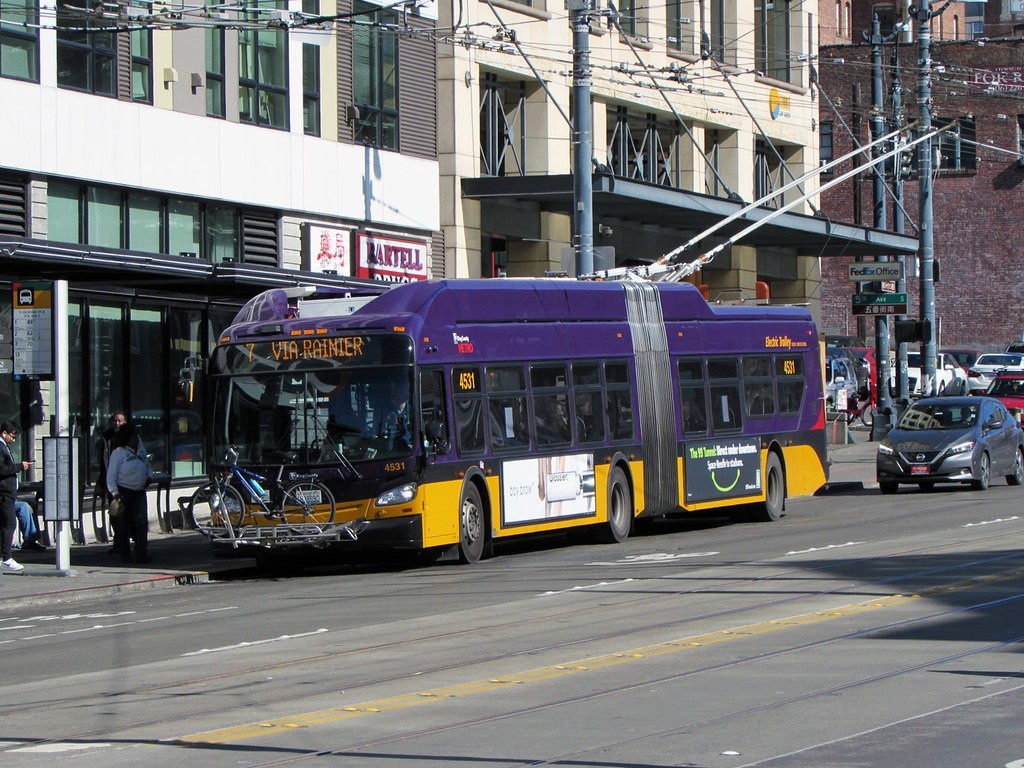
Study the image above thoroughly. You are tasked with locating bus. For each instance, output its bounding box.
[177,121,957,566]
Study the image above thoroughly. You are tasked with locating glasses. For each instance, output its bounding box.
[7,432,16,439]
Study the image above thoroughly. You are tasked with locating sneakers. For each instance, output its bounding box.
[1,558,24,571]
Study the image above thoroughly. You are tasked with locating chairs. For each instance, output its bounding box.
[966,356,974,363]
[934,412,952,424]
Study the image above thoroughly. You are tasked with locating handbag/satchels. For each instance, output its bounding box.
[109,497,125,516]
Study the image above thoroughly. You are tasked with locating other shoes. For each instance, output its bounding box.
[21,542,46,550]
[122,555,135,564]
[136,554,154,564]
[107,546,121,553]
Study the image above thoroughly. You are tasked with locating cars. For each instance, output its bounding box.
[894,341,1024,428]
[876,397,1024,494]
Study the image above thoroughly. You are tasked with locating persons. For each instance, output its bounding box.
[959,405,975,427]
[14,502,47,551]
[0,421,34,571]
[97,410,136,541]
[108,422,151,561]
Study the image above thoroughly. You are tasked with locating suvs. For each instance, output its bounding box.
[823,336,876,413]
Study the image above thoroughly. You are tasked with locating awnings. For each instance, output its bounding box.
[0,236,208,281]
[212,260,399,328]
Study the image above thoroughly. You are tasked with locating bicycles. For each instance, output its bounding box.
[189,443,335,539]
[834,391,876,427]
